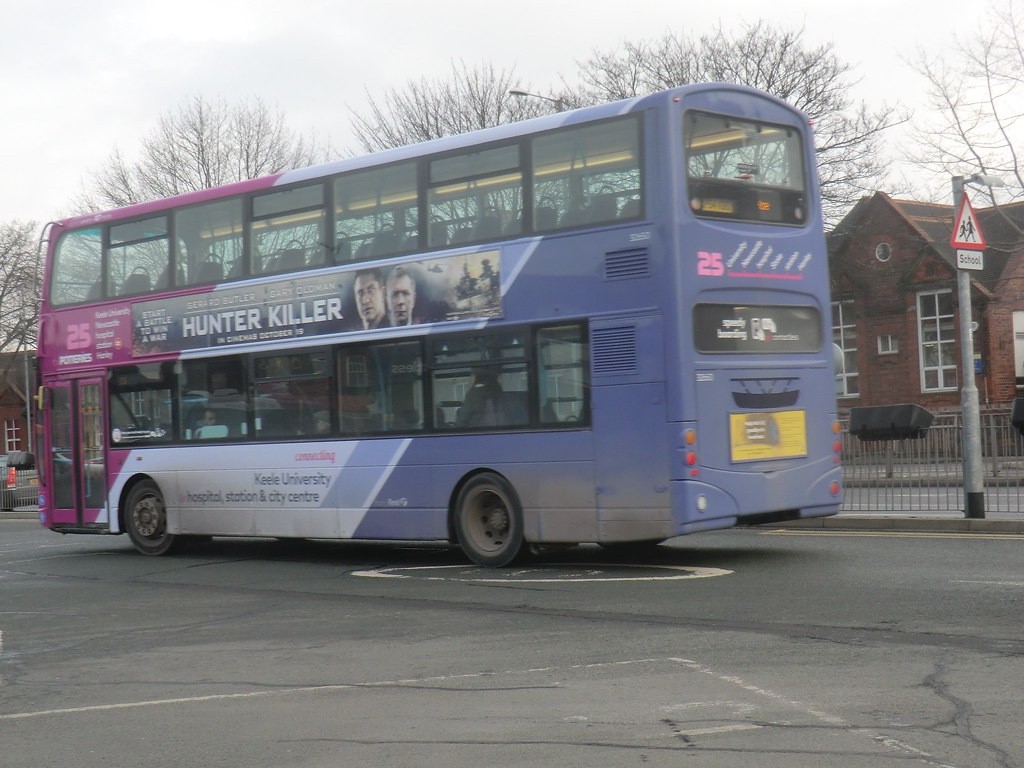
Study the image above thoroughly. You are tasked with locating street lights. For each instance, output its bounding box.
[951,173,1005,517]
[508,91,574,112]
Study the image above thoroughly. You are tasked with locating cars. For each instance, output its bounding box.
[0,454,40,511]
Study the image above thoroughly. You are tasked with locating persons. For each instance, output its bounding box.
[354,267,425,333]
[202,409,218,426]
[402,409,420,428]
[456,366,503,427]
[314,410,331,434]
[437,407,451,428]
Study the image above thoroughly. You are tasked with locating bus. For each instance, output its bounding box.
[34,82,843,568]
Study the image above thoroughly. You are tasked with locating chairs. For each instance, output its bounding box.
[80,185,643,300]
